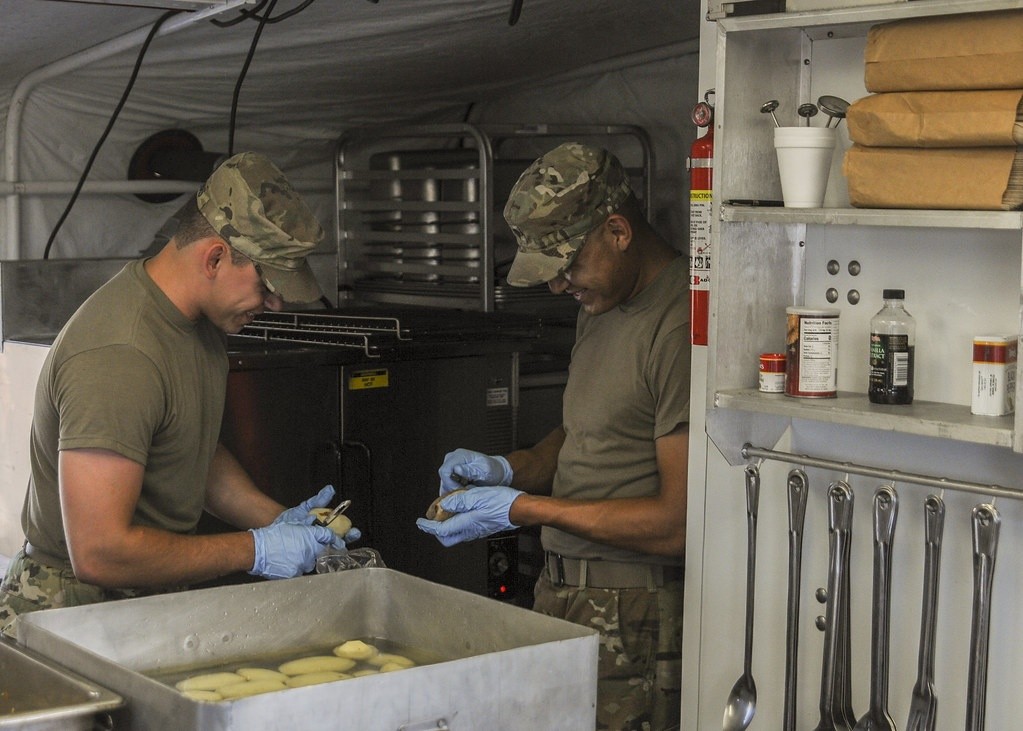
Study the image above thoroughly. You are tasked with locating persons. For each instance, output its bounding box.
[0,150,363,645]
[411,142,689,731]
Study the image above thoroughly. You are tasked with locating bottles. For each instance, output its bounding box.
[869,289,915,403]
[758,353,785,392]
[971,334,1018,416]
[785,305,840,397]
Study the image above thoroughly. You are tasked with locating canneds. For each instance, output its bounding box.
[784,305,840,399]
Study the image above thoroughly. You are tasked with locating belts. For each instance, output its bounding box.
[26,542,65,570]
[547,553,677,588]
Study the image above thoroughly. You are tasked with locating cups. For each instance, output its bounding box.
[774,127,837,208]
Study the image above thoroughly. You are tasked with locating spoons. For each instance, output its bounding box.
[720,464,760,731]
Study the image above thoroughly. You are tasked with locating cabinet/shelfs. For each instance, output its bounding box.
[684,0,1023,731]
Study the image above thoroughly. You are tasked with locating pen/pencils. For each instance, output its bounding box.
[723,199,784,207]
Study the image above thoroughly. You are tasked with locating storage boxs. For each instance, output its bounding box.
[330,120,658,314]
[0,633,127,731]
[15,567,601,731]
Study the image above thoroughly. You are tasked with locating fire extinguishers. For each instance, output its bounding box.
[687,89,715,345]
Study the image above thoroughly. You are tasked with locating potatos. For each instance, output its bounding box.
[175,640,414,703]
[426,490,466,522]
[308,508,351,537]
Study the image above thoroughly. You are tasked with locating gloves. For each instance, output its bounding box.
[415,486,527,548]
[438,448,513,498]
[246,484,362,580]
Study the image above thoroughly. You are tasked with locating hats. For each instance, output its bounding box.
[197,151,327,306]
[503,140,633,287]
